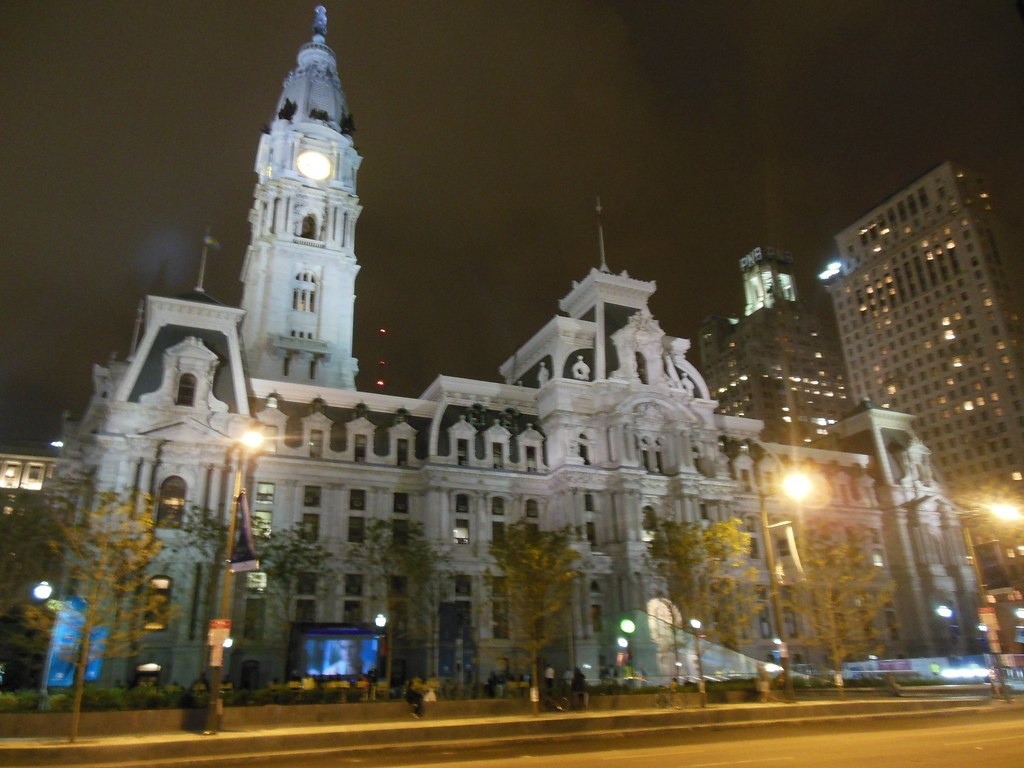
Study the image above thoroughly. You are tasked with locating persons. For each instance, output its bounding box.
[564,666,585,711]
[671,677,680,692]
[488,669,525,700]
[406,681,424,719]
[544,663,555,697]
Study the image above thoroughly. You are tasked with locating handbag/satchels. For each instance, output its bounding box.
[405,681,420,705]
[759,681,770,692]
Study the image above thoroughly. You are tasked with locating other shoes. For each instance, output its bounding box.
[412,713,420,720]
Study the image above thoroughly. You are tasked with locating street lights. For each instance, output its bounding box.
[757,473,817,703]
[375,614,392,699]
[203,429,266,733]
[690,620,707,706]
